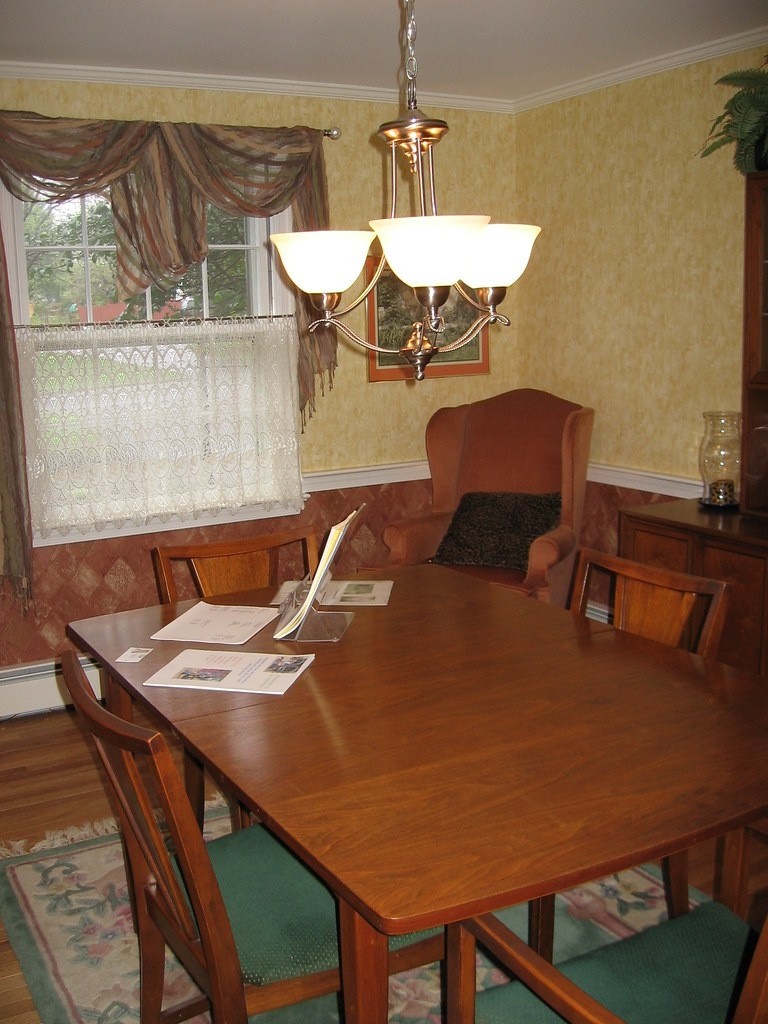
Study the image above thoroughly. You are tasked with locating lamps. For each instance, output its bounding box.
[269,0,543,382]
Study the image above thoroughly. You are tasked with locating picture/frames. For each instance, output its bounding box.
[363,254,491,382]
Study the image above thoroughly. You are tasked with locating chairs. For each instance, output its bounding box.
[152,530,320,837]
[357,388,596,608]
[61,648,447,1023]
[446,815,765,1024]
[528,546,733,965]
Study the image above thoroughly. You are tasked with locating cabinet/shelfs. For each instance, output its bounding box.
[619,171,768,683]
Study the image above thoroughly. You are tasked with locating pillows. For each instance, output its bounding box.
[432,492,562,571]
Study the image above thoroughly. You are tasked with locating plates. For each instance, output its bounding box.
[697,497,741,511]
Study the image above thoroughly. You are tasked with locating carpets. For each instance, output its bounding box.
[0,789,717,1023]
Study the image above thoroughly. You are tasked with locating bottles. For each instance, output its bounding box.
[700,411,741,506]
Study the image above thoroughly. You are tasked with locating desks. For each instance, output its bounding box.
[65,563,767,1024]
